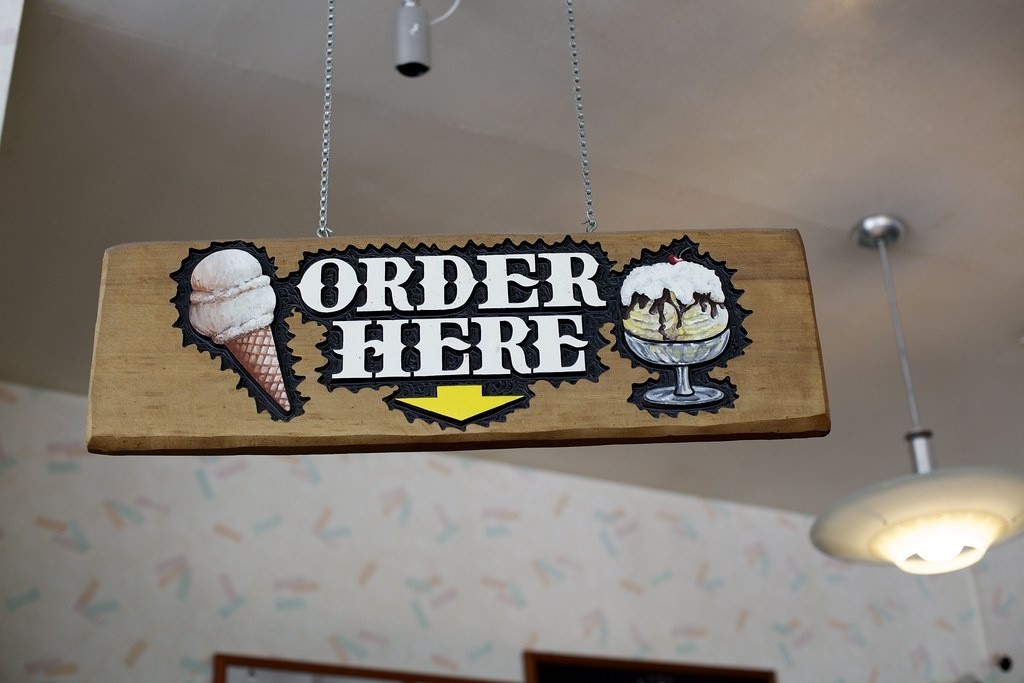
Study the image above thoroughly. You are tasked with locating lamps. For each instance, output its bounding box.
[808,215,1024,576]
